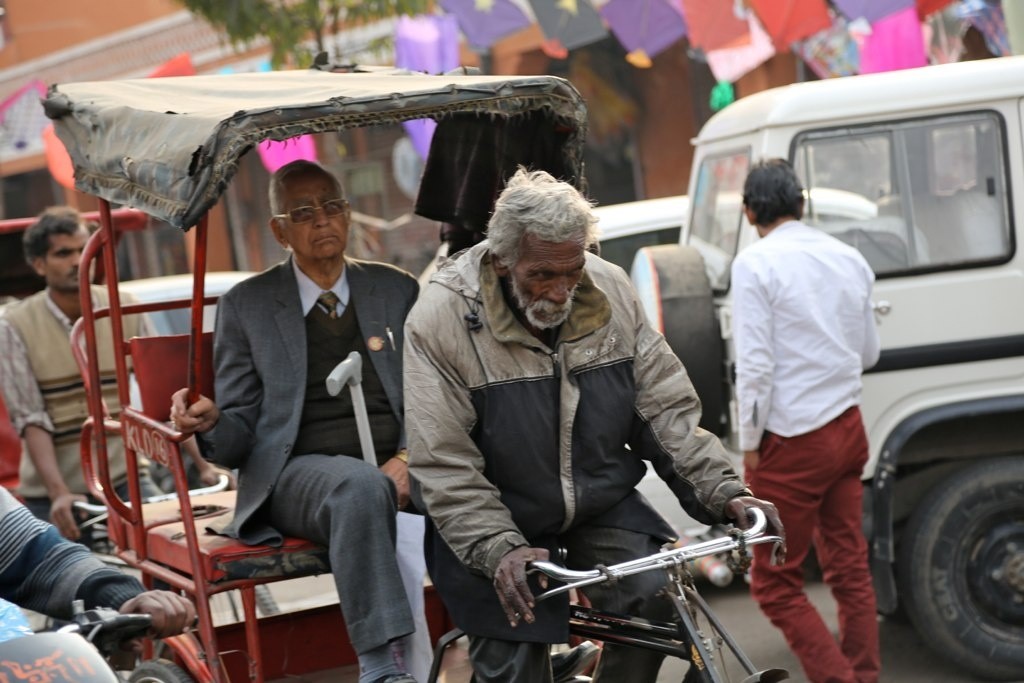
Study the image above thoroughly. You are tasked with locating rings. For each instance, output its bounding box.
[397,504,401,509]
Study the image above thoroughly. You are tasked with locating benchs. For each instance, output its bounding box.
[124,331,328,580]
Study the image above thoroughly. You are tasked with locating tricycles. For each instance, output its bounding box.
[43,66,791,683]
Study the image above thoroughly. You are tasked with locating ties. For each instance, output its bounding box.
[315,290,341,319]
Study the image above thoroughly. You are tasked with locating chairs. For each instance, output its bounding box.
[946,188,1002,260]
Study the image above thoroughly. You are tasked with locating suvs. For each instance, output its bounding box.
[628,50,1024,680]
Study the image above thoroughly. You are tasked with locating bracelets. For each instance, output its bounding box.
[394,451,407,464]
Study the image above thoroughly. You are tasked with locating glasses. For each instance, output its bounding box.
[274,198,350,224]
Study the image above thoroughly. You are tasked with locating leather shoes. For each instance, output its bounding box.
[357,640,417,683]
[551,639,600,681]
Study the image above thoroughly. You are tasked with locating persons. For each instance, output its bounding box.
[0,160,422,682]
[732,158,880,683]
[401,162,788,683]
